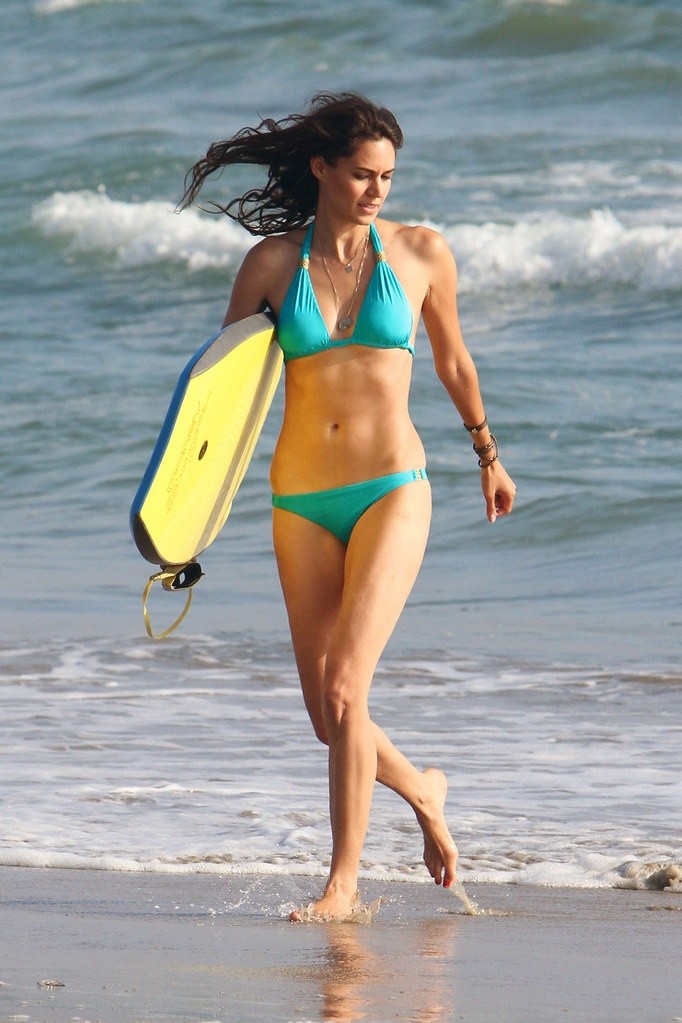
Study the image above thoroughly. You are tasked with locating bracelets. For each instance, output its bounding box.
[473,433,499,467]
[462,415,487,434]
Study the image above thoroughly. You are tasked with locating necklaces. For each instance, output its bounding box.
[312,227,370,331]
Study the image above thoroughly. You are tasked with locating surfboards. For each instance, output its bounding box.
[131,311,288,572]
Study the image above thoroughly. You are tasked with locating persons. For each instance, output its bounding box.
[161,89,518,920]
